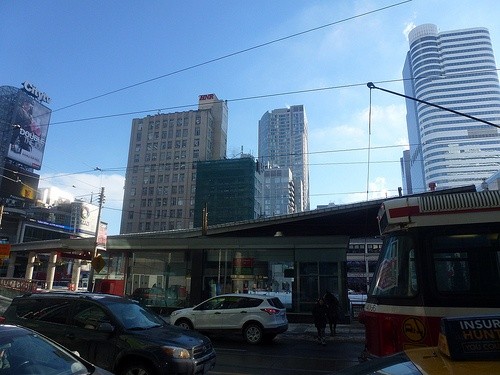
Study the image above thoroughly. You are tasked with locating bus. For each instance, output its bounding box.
[359,190,498,365]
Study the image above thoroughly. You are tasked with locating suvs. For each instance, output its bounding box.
[168,293,288,344]
[6,288,209,375]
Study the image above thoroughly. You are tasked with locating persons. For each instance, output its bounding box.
[312,298,329,345]
[324,288,339,337]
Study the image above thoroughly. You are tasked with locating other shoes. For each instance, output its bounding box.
[318,337,326,345]
[330,332,337,336]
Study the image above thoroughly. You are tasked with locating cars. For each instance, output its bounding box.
[340,316,500,375]
[0,323,114,375]
